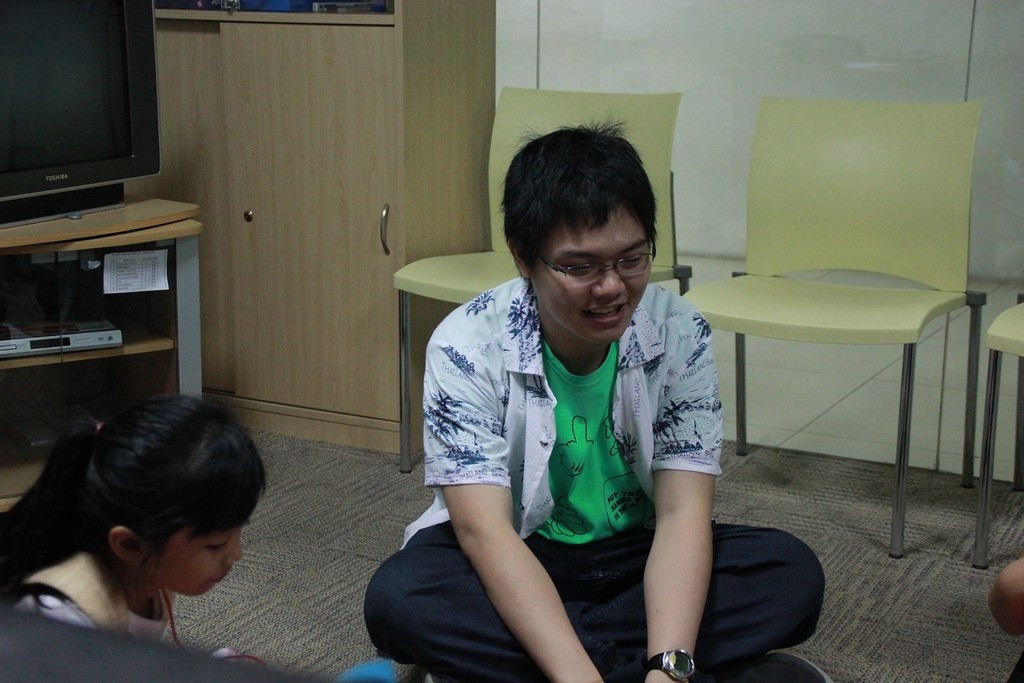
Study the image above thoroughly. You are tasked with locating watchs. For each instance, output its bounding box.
[644,649,694,683]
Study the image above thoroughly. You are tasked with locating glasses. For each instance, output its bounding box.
[537,239,659,287]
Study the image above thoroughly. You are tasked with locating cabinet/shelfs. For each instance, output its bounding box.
[0,193,205,514]
[151,0,495,455]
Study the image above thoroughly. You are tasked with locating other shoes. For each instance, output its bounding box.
[724,652,832,683]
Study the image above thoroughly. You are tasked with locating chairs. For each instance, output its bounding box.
[683,99,985,559]
[393,87,694,473]
[970,294,1024,569]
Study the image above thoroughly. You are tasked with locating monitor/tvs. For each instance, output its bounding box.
[0,0,162,230]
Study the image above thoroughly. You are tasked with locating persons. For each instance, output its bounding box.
[363,126,834,683]
[0,394,267,665]
